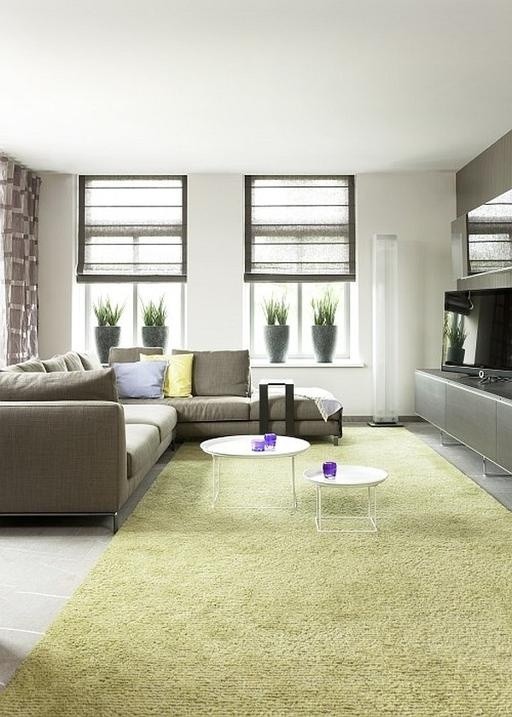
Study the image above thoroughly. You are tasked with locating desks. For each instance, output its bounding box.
[198,432,311,513]
[301,461,387,535]
[257,375,297,438]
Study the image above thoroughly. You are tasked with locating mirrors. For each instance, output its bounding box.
[463,186,512,276]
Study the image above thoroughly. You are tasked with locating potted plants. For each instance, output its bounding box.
[93,296,124,363]
[139,297,171,349]
[444,308,469,364]
[309,291,342,364]
[260,295,294,365]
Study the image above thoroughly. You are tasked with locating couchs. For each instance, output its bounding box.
[0,343,345,533]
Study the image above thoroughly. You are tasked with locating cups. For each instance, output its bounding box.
[264,433,276,448]
[323,461,336,478]
[251,439,264,450]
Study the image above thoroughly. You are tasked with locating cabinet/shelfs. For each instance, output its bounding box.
[416,364,512,478]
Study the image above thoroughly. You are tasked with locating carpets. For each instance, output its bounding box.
[0,420,512,717]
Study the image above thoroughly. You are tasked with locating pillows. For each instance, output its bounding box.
[141,350,194,396]
[113,360,171,399]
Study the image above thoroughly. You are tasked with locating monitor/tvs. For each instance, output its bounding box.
[440,287,512,383]
